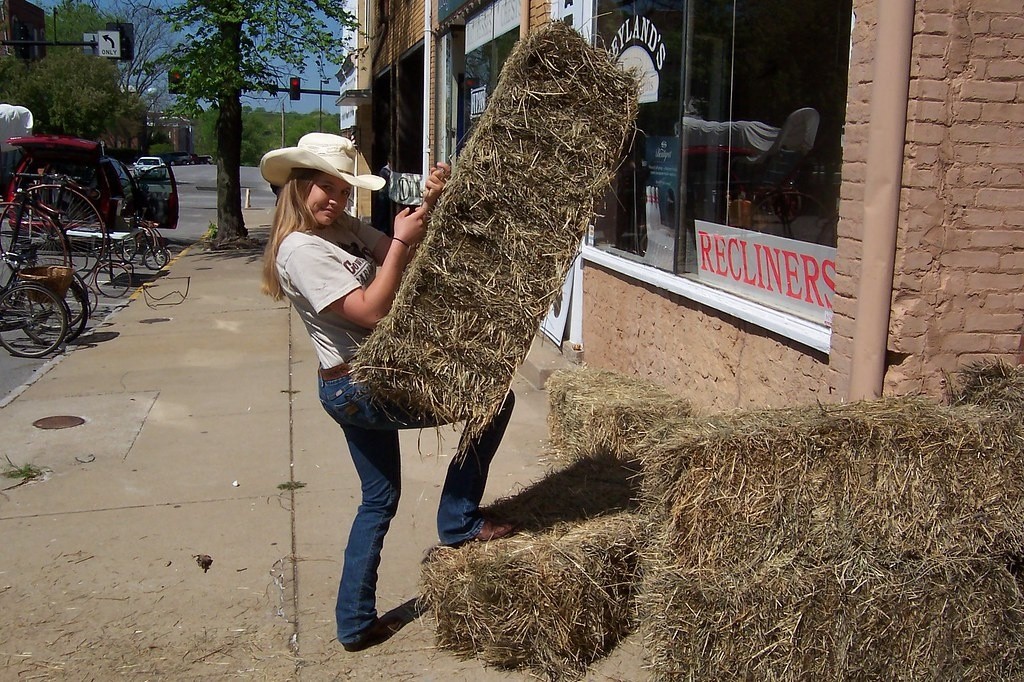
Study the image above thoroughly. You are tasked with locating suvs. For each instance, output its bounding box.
[171,151,214,165]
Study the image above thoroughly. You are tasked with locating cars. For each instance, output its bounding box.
[133,156,167,181]
[7,136,180,257]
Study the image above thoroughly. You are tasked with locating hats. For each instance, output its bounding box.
[260,133,386,191]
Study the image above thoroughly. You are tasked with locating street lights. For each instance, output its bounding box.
[319,79,329,132]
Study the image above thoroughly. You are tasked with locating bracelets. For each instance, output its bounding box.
[393,238,409,248]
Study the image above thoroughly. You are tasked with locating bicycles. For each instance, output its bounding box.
[0,173,107,358]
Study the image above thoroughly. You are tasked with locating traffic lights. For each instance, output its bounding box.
[458,76,481,115]
[168,70,186,95]
[289,76,302,101]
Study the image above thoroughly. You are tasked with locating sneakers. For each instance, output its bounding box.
[446,518,515,547]
[343,616,402,652]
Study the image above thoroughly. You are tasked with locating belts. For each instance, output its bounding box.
[317,361,350,381]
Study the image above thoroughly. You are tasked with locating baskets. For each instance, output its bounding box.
[18,265,73,303]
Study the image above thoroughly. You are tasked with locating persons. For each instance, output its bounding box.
[259,131,522,653]
